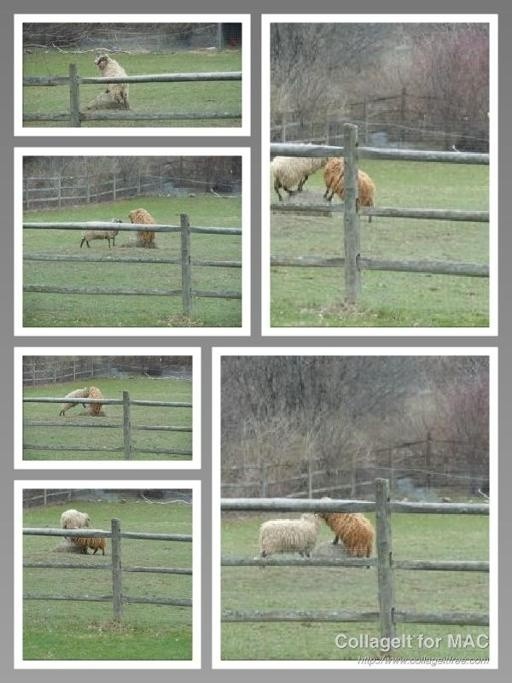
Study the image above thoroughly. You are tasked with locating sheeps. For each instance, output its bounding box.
[315,496,376,569]
[80,218,124,248]
[71,535,108,555]
[126,206,156,246]
[269,142,327,202]
[60,508,93,542]
[258,510,323,560]
[321,154,377,224]
[58,385,88,416]
[93,52,130,111]
[87,384,104,416]
